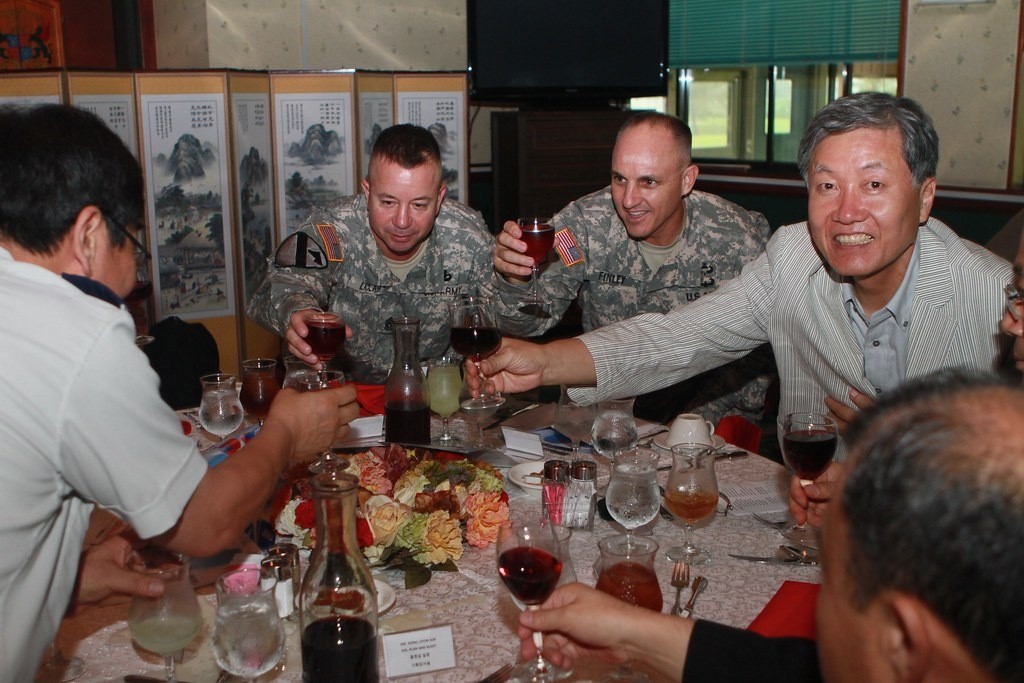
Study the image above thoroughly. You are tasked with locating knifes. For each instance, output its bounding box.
[728,554,819,566]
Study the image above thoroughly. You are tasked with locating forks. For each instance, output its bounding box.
[753,513,787,530]
[668,561,690,615]
[476,661,515,683]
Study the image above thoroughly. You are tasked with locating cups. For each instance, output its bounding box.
[664,414,714,445]
[518,523,577,589]
[199,311,348,439]
[211,568,287,683]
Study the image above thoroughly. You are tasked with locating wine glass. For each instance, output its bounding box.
[781,412,838,541]
[666,441,719,566]
[606,449,661,552]
[448,296,506,409]
[591,397,640,497]
[518,215,556,305]
[552,384,595,464]
[427,356,462,445]
[593,535,662,683]
[495,520,576,683]
[124,546,202,682]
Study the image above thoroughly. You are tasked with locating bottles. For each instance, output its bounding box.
[384,317,431,445]
[541,459,596,532]
[299,473,379,683]
[261,544,302,618]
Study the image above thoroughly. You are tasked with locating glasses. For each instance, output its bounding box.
[100,211,152,271]
[1001,283,1024,321]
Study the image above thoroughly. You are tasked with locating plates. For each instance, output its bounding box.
[295,576,395,613]
[653,430,725,451]
[509,462,545,489]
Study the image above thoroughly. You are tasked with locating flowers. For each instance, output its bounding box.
[272,441,512,591]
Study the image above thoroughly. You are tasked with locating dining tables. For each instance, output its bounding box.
[41,372,853,683]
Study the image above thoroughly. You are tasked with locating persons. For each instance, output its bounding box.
[245,123,544,402]
[516,368,1024,683]
[490,110,772,431]
[464,92,1013,473]
[788,213,1024,527]
[0,103,360,683]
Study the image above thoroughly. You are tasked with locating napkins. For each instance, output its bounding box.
[746,580,822,641]
[350,381,424,419]
